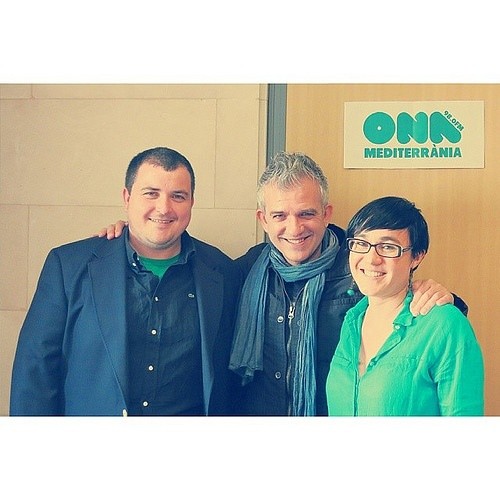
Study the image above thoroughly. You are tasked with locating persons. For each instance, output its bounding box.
[324,196,487,415]
[6,146,243,416]
[92,151,469,417]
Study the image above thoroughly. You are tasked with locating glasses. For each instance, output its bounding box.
[346,236,416,260]
